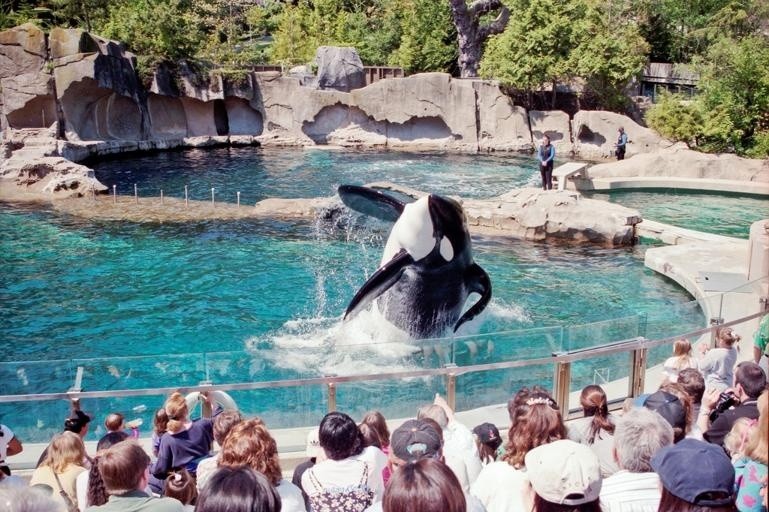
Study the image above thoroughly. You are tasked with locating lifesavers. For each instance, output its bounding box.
[185,391,237,420]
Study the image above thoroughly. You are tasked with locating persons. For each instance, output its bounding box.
[537,135,555,189]
[615,126,626,160]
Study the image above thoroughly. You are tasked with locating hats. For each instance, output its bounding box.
[523,442,605,506]
[649,436,741,508]
[633,388,688,439]
[390,418,445,464]
[473,423,502,448]
[66,410,95,429]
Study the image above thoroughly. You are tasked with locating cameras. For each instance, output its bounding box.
[716,393,735,413]
